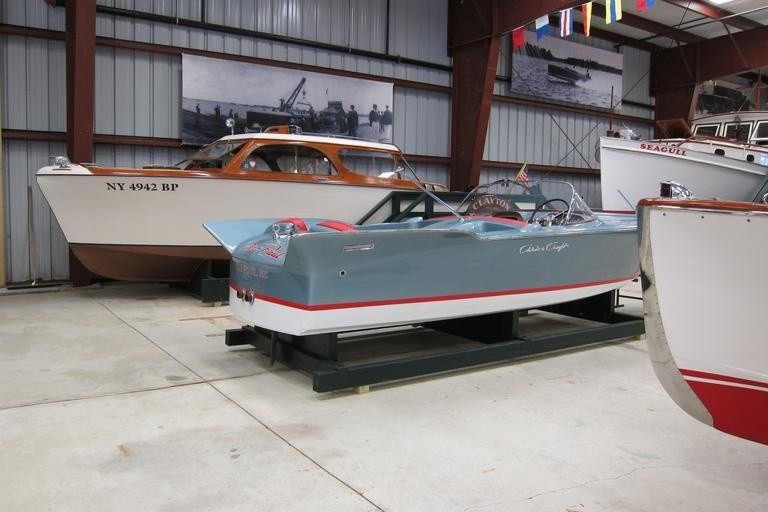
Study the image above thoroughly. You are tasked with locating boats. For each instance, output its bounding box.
[182,78,348,134]
[548,63,593,82]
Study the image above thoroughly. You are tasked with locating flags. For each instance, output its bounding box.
[637,1,654,11]
[606,0,622,25]
[581,2,593,38]
[518,162,530,198]
[557,8,574,39]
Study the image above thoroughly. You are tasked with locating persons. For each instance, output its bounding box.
[346,104,359,138]
[586,69,591,79]
[368,104,379,143]
[383,106,393,139]
